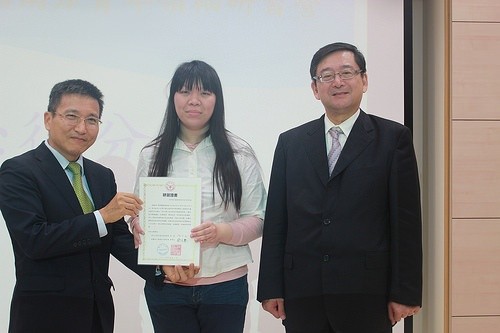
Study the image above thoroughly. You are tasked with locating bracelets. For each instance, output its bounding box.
[131,217,138,223]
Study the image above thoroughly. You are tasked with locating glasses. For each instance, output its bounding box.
[55,111,102,128]
[313,69,362,82]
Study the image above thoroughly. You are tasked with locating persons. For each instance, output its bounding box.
[126,59,268,333]
[257,42,422,333]
[0,79,200,333]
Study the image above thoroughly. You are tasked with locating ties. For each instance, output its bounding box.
[68,162,94,215]
[328,127,343,177]
